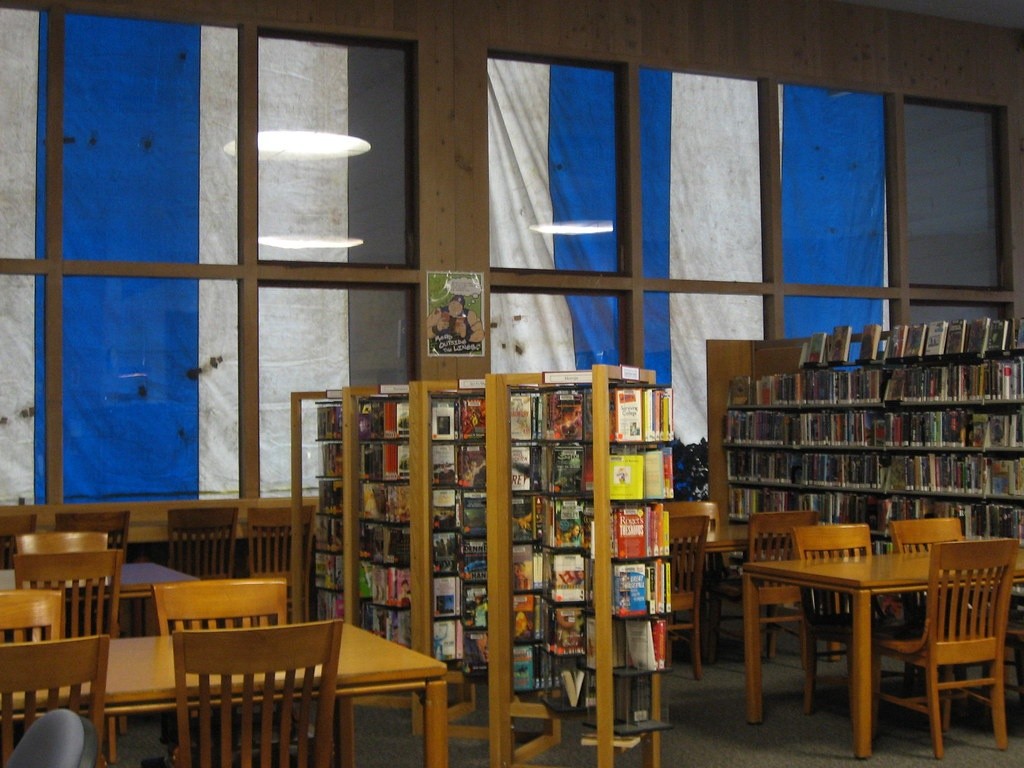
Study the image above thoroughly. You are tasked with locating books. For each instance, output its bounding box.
[720,315,1024,617]
[312,380,674,728]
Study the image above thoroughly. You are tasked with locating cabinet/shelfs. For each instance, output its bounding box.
[291,365,671,768]
[705,332,1024,524]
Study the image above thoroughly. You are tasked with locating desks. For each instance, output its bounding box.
[742,549,1024,759]
[0,563,202,636]
[0,624,447,768]
[669,524,786,553]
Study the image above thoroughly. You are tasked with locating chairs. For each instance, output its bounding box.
[0,505,343,768]
[665,501,1024,758]
